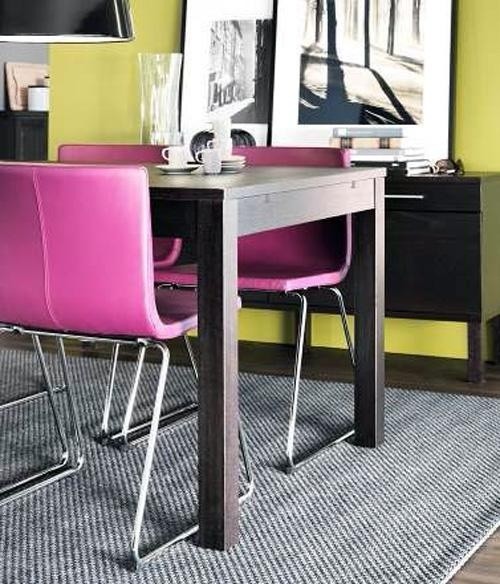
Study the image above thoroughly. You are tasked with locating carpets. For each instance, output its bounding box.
[0,347,500,584]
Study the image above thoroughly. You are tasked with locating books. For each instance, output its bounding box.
[333,124,432,174]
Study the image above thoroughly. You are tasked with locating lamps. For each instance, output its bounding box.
[0,0,135,44]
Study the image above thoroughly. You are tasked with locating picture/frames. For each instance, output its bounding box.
[179,0,275,148]
[270,0,453,163]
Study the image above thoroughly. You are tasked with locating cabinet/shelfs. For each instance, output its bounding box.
[241,172,500,385]
[0,110,49,161]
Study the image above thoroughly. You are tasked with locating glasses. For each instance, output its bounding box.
[435,158,466,174]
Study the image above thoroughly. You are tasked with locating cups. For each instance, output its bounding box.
[195,150,222,175]
[207,120,234,158]
[160,146,190,166]
[26,85,49,113]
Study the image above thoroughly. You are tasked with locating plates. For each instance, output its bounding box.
[220,155,248,174]
[155,164,200,175]
